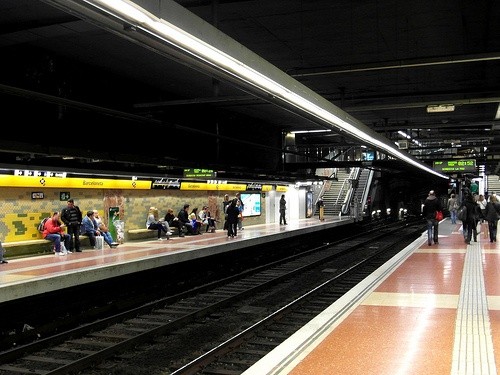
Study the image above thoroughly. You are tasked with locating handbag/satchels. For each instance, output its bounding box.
[96,235,104,249]
[53,241,68,255]
[496,212,500,220]
[436,211,443,220]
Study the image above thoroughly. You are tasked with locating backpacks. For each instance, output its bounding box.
[457,205,467,221]
[38,217,48,232]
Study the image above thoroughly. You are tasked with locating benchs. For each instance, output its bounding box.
[128,227,180,233]
[1,235,90,259]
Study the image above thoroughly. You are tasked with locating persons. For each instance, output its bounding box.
[61,199,82,252]
[459,192,500,244]
[200,206,221,233]
[188,207,202,235]
[165,208,185,237]
[222,192,244,239]
[178,204,194,235]
[0,241,8,264]
[279,194,288,225]
[42,211,73,256]
[448,193,459,224]
[318,196,325,220]
[79,210,101,248]
[424,190,443,246]
[91,210,119,248]
[146,207,174,241]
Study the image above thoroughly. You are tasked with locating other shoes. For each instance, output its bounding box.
[55,251,65,256]
[67,250,73,254]
[110,242,119,248]
[165,232,172,235]
[158,237,162,240]
